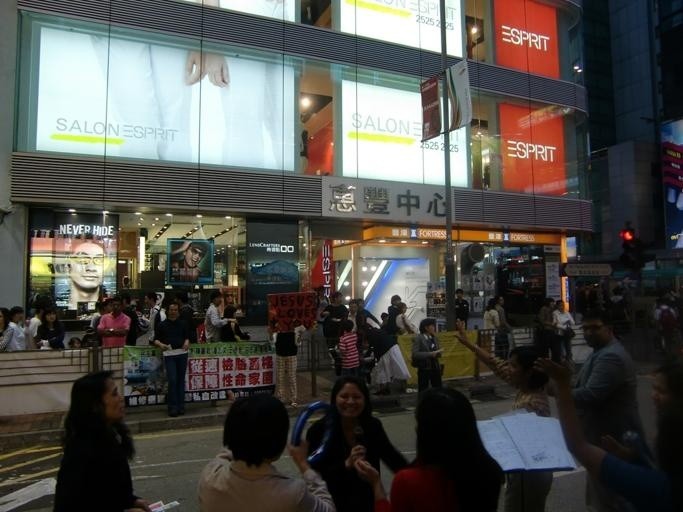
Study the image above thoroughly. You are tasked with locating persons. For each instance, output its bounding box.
[606,286,633,337]
[168,240,212,285]
[89,0,207,166]
[313,284,418,401]
[532,357,683,512]
[454,319,553,512]
[197,392,337,511]
[143,292,252,347]
[651,286,683,353]
[68,294,150,349]
[207,0,293,170]
[305,377,412,511]
[455,288,470,331]
[537,297,576,360]
[52,370,153,512]
[353,387,506,512]
[153,299,194,416]
[412,317,446,391]
[548,305,644,446]
[1,293,66,353]
[484,296,507,330]
[53,213,116,312]
[271,319,303,407]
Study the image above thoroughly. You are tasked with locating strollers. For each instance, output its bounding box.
[328,347,374,381]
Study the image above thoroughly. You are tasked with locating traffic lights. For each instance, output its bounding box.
[621,228,634,247]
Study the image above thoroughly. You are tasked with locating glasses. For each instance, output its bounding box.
[191,248,203,257]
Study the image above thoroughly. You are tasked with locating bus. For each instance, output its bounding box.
[566,267,682,320]
